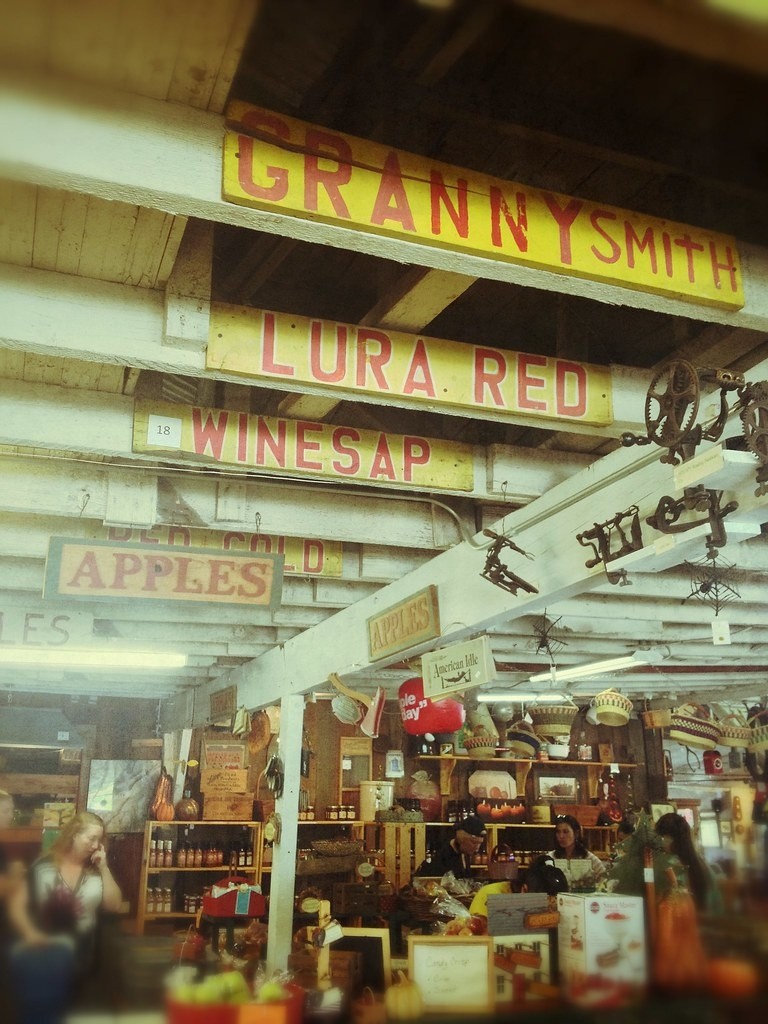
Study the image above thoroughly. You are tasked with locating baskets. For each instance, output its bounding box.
[595,688,634,727]
[640,696,671,729]
[526,689,579,736]
[505,720,542,756]
[719,714,751,748]
[670,703,721,749]
[352,987,385,1024]
[463,725,497,756]
[399,890,475,922]
[487,844,518,880]
[748,711,768,753]
[312,837,366,857]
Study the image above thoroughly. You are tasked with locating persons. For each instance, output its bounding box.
[542,814,607,888]
[410,815,488,884]
[471,855,569,934]
[655,813,723,918]
[617,825,637,845]
[0,811,122,1024]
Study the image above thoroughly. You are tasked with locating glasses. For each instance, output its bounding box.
[556,815,572,824]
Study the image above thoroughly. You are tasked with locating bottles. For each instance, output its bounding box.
[537,743,549,760]
[446,798,474,822]
[298,806,315,821]
[531,796,551,824]
[425,848,550,866]
[146,840,253,914]
[325,805,356,820]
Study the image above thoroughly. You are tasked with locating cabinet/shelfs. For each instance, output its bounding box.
[137,753,635,938]
